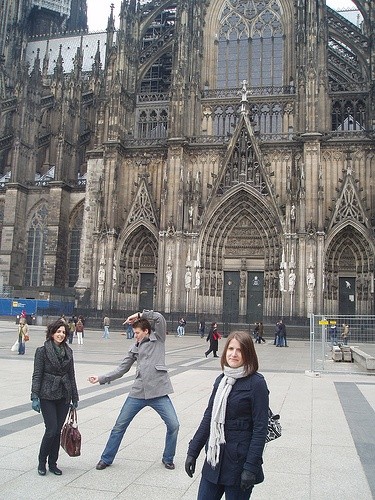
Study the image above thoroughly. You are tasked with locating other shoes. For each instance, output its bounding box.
[21,353,24,355]
[205,353,207,358]
[18,353,21,355]
[214,355,219,357]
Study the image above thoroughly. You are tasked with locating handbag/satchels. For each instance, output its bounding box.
[24,336,29,341]
[265,408,282,443]
[61,408,81,456]
[212,328,220,341]
[11,339,19,352]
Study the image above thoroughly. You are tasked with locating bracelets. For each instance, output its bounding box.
[137,311,141,320]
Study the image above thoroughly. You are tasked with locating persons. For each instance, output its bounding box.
[288,269,296,292]
[184,267,192,289]
[177,317,186,337]
[341,322,349,345]
[253,322,265,344]
[195,269,201,289]
[184,331,269,500]
[98,265,105,284]
[126,315,134,339]
[279,269,284,291]
[329,324,337,350]
[102,314,110,339]
[273,320,288,347]
[31,320,80,475]
[17,318,30,355]
[205,322,221,358]
[86,309,180,470]
[59,314,86,344]
[112,266,117,285]
[166,266,173,286]
[307,269,316,290]
[198,319,205,338]
[20,310,26,318]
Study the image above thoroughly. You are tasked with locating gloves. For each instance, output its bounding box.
[73,401,78,408]
[32,399,40,413]
[241,470,256,489]
[185,456,196,478]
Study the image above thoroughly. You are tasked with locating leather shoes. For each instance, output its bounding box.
[162,459,174,469]
[38,466,46,475]
[49,466,62,475]
[96,460,108,469]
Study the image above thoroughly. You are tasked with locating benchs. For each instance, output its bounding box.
[350,345,375,370]
